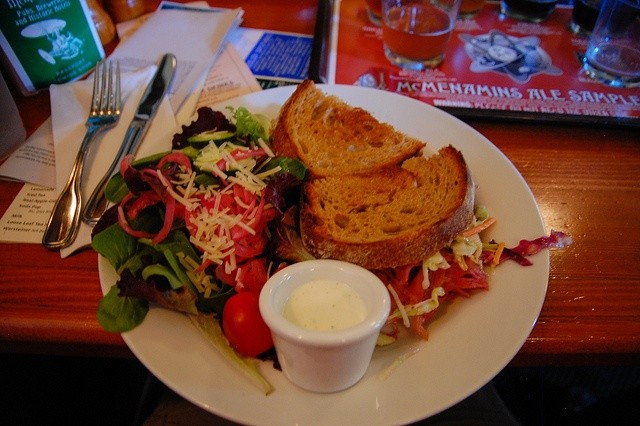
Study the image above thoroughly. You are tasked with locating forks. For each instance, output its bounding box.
[42,60,121,250]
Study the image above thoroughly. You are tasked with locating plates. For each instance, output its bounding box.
[98,84,549,426]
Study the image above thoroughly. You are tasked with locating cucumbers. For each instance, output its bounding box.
[187,129,236,149]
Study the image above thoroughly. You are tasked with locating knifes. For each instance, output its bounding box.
[82,53,177,227]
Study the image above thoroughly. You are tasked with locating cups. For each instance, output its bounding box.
[258,258,392,394]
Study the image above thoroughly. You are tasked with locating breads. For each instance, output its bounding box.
[299,143,475,269]
[272,79,427,180]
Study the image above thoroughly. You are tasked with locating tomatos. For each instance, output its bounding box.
[223,292,273,357]
[185,183,275,292]
